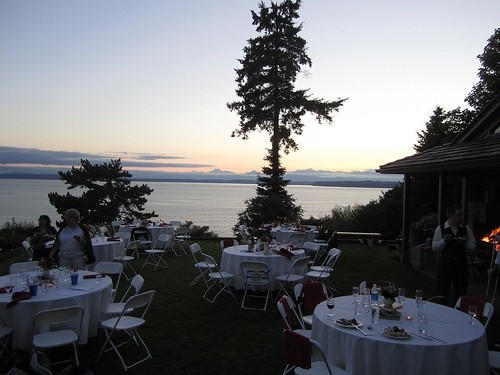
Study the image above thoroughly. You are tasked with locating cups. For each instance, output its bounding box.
[71,274,78,285]
[29,283,38,296]
[355,298,361,315]
[96,270,103,283]
[398,289,405,303]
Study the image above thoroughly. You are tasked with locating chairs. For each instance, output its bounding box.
[0,221,193,375]
[189,222,500,375]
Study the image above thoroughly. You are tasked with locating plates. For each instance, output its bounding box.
[334,318,363,327]
[383,328,412,338]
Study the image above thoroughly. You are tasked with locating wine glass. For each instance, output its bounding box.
[468,303,478,324]
[326,299,335,319]
[5,280,12,297]
[352,287,359,303]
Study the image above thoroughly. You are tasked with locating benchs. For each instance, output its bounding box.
[337,232,381,244]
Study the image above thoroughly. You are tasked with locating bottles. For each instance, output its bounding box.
[362,288,372,328]
[360,281,366,295]
[419,315,429,337]
[371,284,378,304]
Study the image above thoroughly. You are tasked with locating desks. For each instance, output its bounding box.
[45,236,124,270]
[119,225,175,249]
[221,244,308,292]
[0,269,113,351]
[264,225,315,245]
[311,295,491,375]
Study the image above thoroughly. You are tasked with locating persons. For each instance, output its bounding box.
[30,215,60,268]
[83,220,150,242]
[49,209,96,271]
[433,206,476,307]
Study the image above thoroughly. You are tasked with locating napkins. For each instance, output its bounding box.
[107,239,120,241]
[108,237,120,239]
[0,286,14,294]
[6,292,32,309]
[83,273,106,279]
[276,248,295,260]
